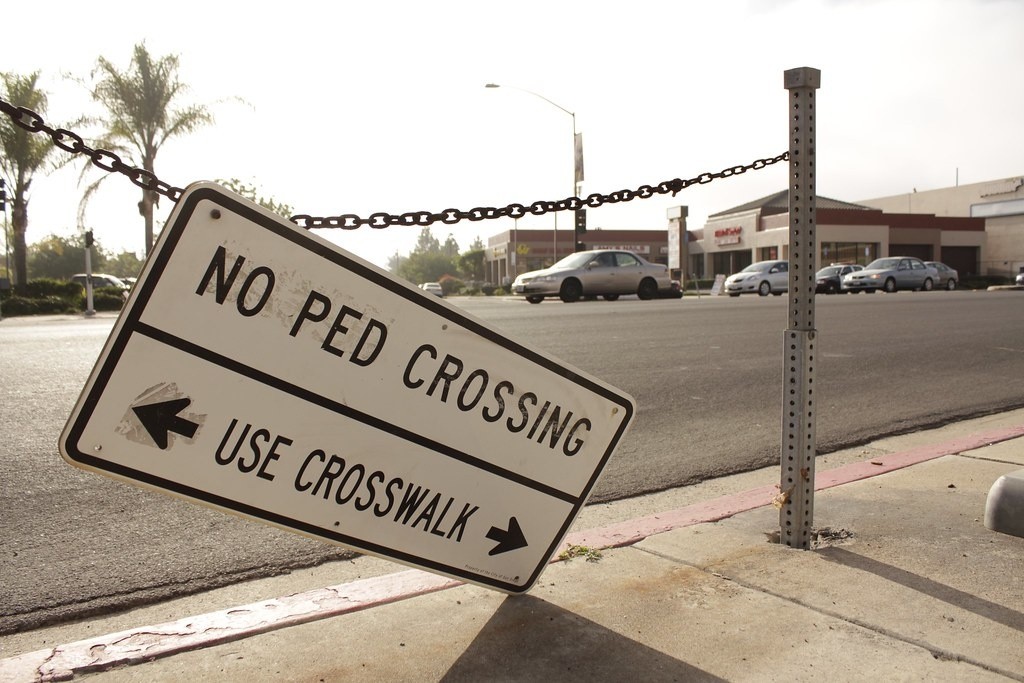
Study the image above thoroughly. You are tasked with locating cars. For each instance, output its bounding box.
[842,256,940,293]
[418,284,424,289]
[422,282,443,297]
[72,274,138,304]
[723,260,788,296]
[814,264,866,294]
[659,279,683,299]
[909,261,959,291]
[510,249,672,303]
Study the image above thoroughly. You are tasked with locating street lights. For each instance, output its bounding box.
[484,82,577,255]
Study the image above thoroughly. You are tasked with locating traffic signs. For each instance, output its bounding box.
[62,182,638,598]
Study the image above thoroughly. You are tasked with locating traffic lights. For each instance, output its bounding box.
[579,208,588,234]
[578,242,587,251]
